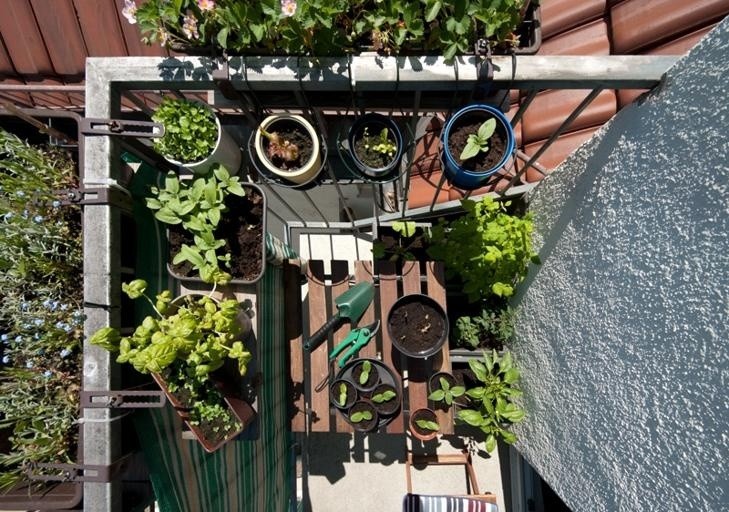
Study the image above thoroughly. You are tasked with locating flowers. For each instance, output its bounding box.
[120,2,348,63]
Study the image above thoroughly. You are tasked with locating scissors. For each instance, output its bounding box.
[328,318,382,370]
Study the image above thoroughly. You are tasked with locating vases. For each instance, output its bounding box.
[386,293,449,358]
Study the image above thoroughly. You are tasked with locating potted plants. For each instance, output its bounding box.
[328,378,358,410]
[370,383,401,416]
[145,353,256,454]
[143,170,267,287]
[149,95,240,178]
[409,407,440,442]
[346,113,403,180]
[365,195,542,303]
[427,371,466,406]
[350,0,545,64]
[450,350,527,453]
[442,104,516,186]
[88,279,253,398]
[255,113,322,184]
[351,360,380,393]
[452,301,524,356]
[348,401,379,432]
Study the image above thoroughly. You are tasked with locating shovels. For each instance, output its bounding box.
[302,281,377,353]
